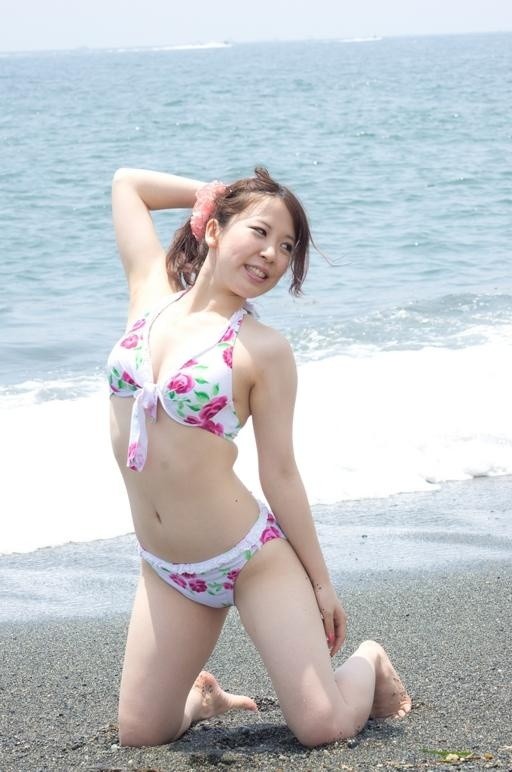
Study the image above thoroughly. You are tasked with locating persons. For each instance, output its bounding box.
[107,165,413,748]
